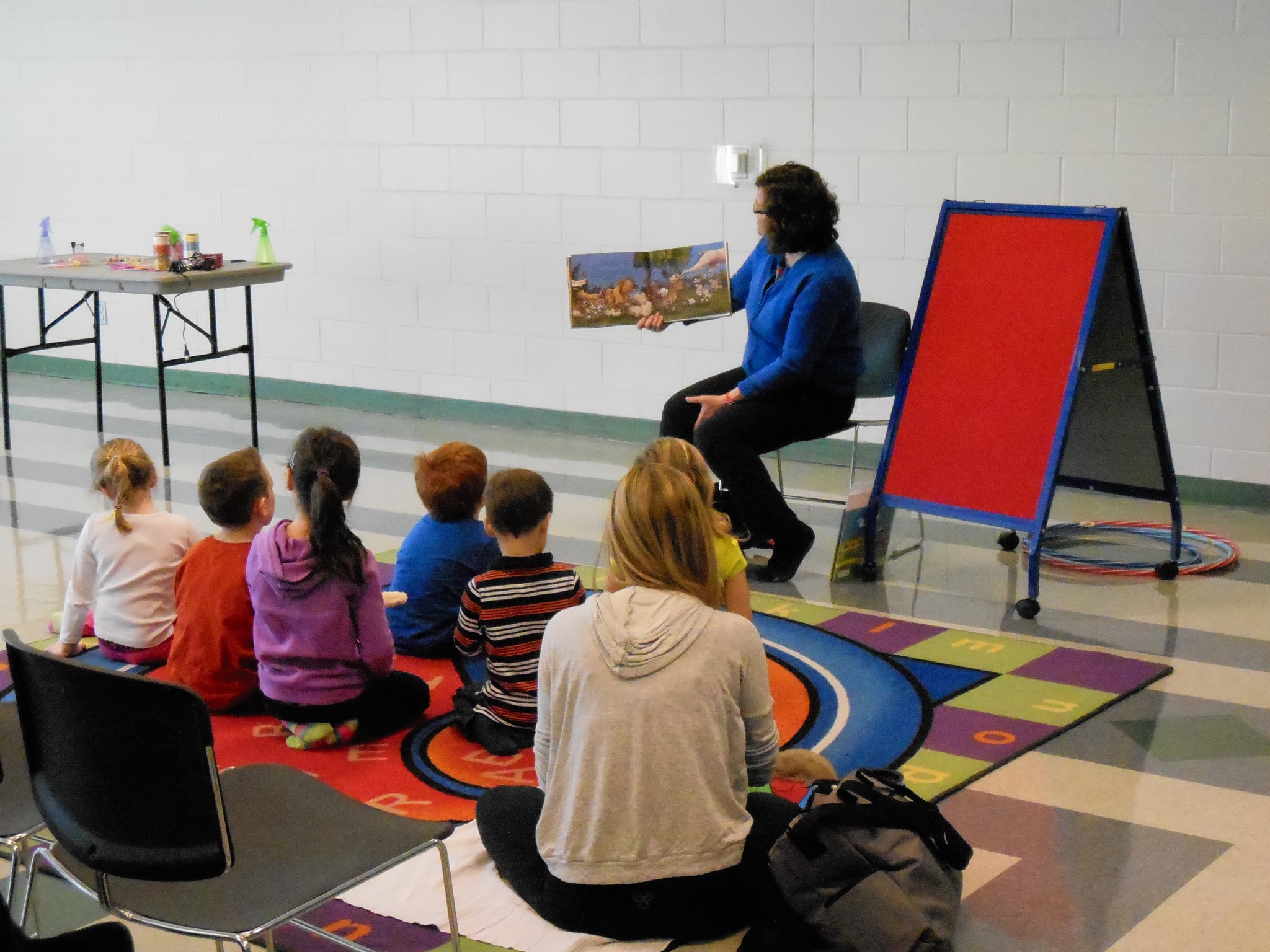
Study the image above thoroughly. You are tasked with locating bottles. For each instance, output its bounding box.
[151,231,200,271]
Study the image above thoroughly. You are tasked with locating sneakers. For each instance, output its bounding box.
[755,520,815,580]
[753,202,767,215]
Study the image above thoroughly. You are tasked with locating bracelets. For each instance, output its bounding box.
[725,392,735,406]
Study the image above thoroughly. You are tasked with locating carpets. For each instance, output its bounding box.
[1,562,1171,952]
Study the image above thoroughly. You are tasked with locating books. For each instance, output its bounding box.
[565,240,732,330]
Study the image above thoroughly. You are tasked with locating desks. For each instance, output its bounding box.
[0,252,294,467]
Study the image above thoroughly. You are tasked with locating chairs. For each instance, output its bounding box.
[760,299,929,578]
[3,625,461,952]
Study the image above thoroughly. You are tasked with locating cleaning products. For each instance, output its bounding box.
[37,217,57,265]
[251,217,275,265]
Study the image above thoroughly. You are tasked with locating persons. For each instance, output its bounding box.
[59,438,206,666]
[244,428,430,750]
[659,162,865,583]
[475,465,801,943]
[452,469,585,754]
[600,439,754,625]
[380,441,502,661]
[164,445,275,716]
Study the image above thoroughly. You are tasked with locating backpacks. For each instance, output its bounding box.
[765,767,974,952]
